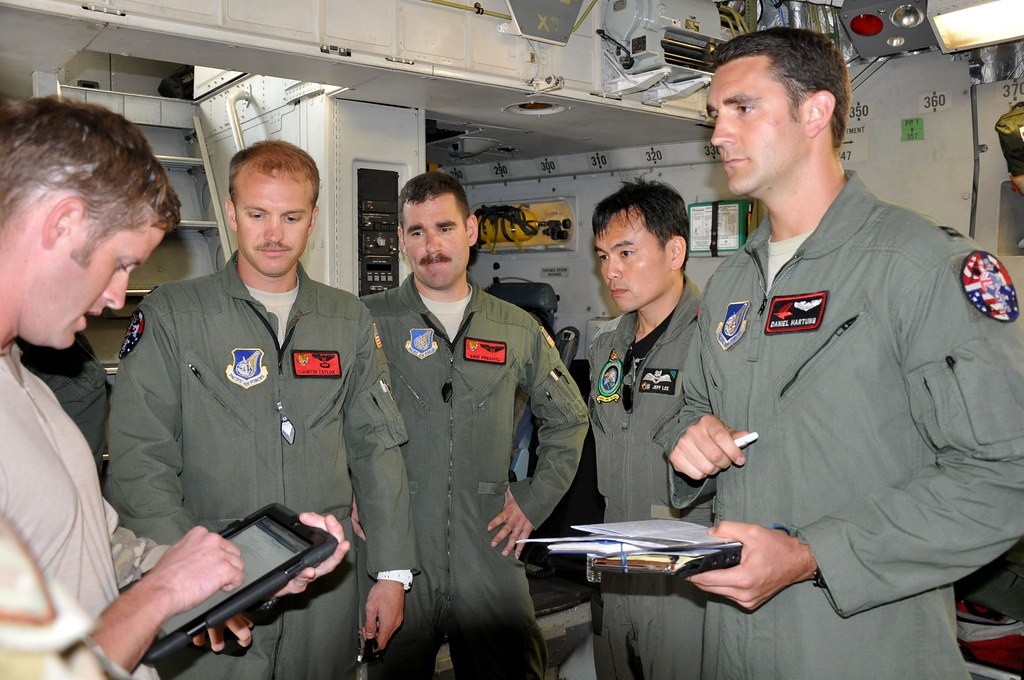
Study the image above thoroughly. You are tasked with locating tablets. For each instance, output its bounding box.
[121,502,338,663]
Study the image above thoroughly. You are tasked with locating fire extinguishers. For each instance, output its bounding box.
[469,204,573,247]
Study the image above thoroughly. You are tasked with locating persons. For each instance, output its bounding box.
[588,180,712,680]
[103,139,421,679]
[14,330,108,474]
[650,26,1024,680]
[0,95,350,680]
[361,172,590,680]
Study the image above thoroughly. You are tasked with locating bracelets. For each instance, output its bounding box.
[377,568,414,591]
[812,568,826,588]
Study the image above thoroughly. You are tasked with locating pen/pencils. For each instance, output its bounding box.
[549,367,570,384]
[379,377,395,401]
[733,430,761,448]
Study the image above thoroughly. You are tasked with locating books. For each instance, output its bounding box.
[518,518,790,579]
